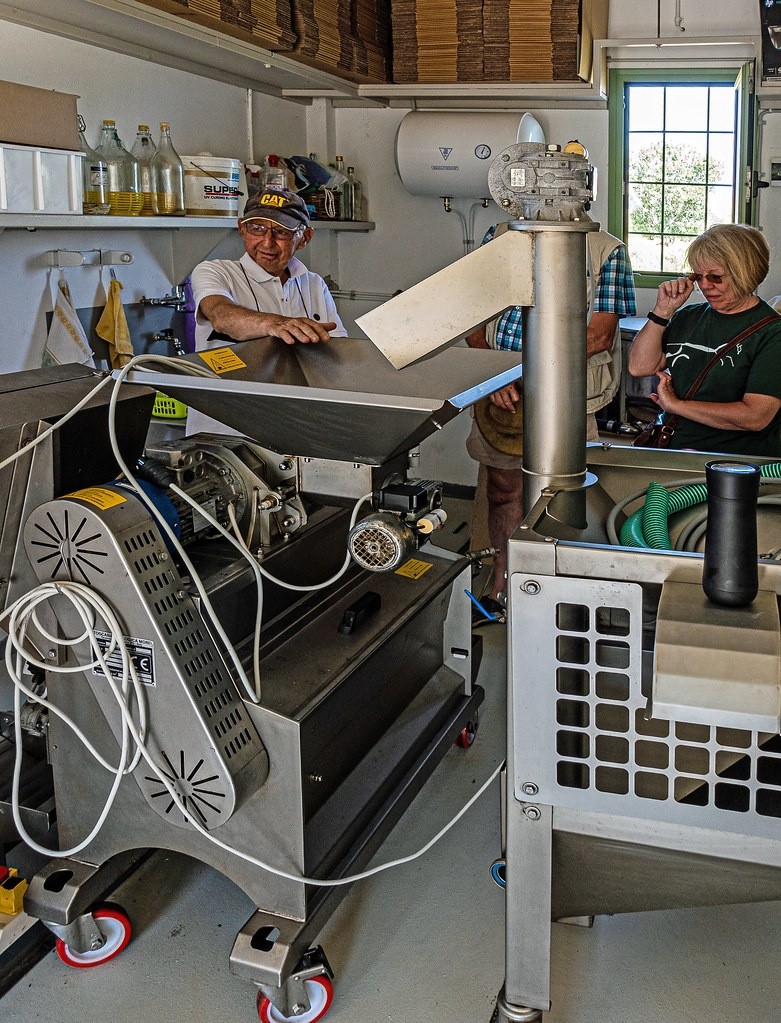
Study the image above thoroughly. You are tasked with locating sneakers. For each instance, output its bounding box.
[471,594,507,630]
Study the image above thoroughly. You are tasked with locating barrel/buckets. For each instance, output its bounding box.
[179,155,241,219]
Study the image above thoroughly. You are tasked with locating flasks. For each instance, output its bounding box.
[701,459,759,607]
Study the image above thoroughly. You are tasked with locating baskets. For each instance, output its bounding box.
[299,190,343,221]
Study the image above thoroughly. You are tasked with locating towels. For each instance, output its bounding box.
[41,279,97,369]
[95,277,136,370]
[182,276,196,354]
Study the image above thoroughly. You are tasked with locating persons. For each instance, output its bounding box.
[465,221,638,627]
[184,189,347,436]
[628,223,781,458]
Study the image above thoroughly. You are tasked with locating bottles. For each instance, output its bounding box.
[149,122,186,217]
[94,119,144,216]
[347,166,363,221]
[335,155,345,176]
[258,154,290,193]
[77,117,112,215]
[129,123,158,216]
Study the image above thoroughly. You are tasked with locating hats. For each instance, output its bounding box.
[240,189,310,231]
[474,379,523,457]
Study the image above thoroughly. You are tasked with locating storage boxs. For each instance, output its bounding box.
[0,81,82,151]
[0,144,87,215]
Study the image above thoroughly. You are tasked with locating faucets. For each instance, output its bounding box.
[155,328,186,357]
[142,285,187,313]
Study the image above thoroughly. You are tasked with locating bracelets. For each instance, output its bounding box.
[647,311,670,327]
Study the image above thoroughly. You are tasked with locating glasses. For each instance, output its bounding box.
[245,222,306,240]
[689,273,732,284]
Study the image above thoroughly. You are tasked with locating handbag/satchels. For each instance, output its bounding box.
[631,414,673,449]
